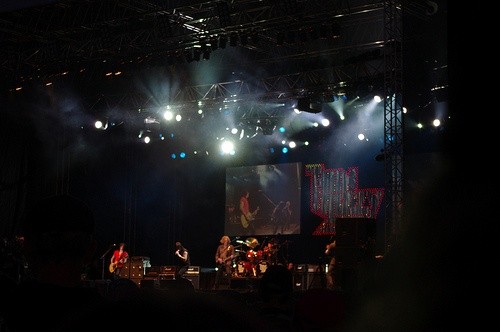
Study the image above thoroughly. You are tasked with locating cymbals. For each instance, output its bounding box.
[245,238,258,247]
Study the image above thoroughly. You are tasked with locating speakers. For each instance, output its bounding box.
[291,273,325,291]
[158,274,200,294]
[119,256,149,288]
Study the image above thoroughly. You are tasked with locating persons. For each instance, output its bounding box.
[0,195,172,332]
[111,243,128,276]
[215,236,234,278]
[325,241,336,289]
[270,200,291,233]
[174,242,190,280]
[252,264,303,332]
[240,192,257,235]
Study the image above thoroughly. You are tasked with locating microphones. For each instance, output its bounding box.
[112,244,116,246]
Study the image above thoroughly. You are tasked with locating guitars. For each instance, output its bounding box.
[212,253,240,274]
[241,206,260,228]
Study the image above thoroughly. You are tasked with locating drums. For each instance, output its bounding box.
[257,246,273,260]
[247,250,257,261]
[237,261,256,277]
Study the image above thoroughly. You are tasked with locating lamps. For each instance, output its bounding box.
[7,52,122,92]
[230,120,277,139]
[274,23,343,47]
[171,27,258,67]
[296,96,323,112]
